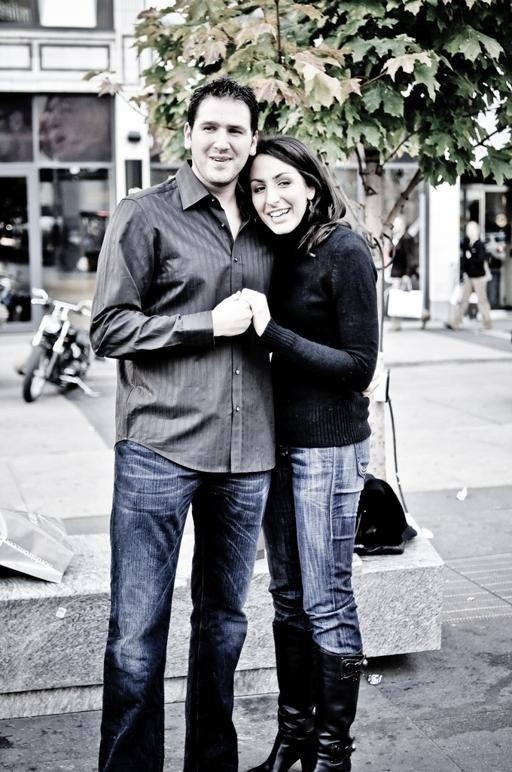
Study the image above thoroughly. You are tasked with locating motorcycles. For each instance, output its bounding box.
[16,288,104,404]
[0,276,33,326]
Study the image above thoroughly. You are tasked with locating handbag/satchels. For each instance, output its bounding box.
[348,473,417,554]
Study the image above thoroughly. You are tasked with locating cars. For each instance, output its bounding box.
[0,203,110,274]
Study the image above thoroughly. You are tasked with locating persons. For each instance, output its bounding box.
[391,220,423,332]
[233,127,392,772]
[441,219,495,333]
[0,201,108,324]
[86,74,286,771]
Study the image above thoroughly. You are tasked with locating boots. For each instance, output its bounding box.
[248,620,319,771]
[307,638,362,772]
